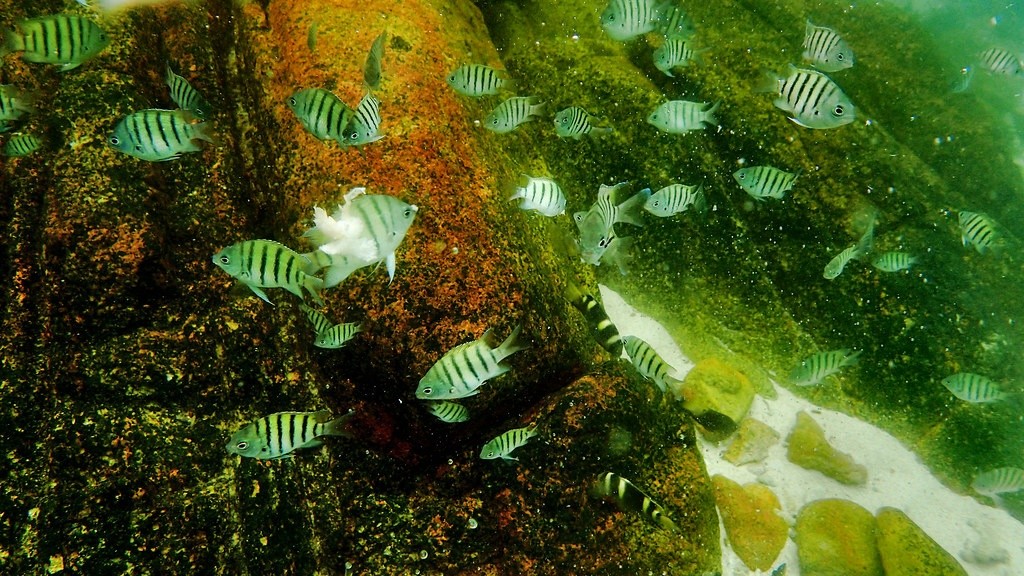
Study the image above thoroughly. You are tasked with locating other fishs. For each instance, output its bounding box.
[0,1,1024,532]
